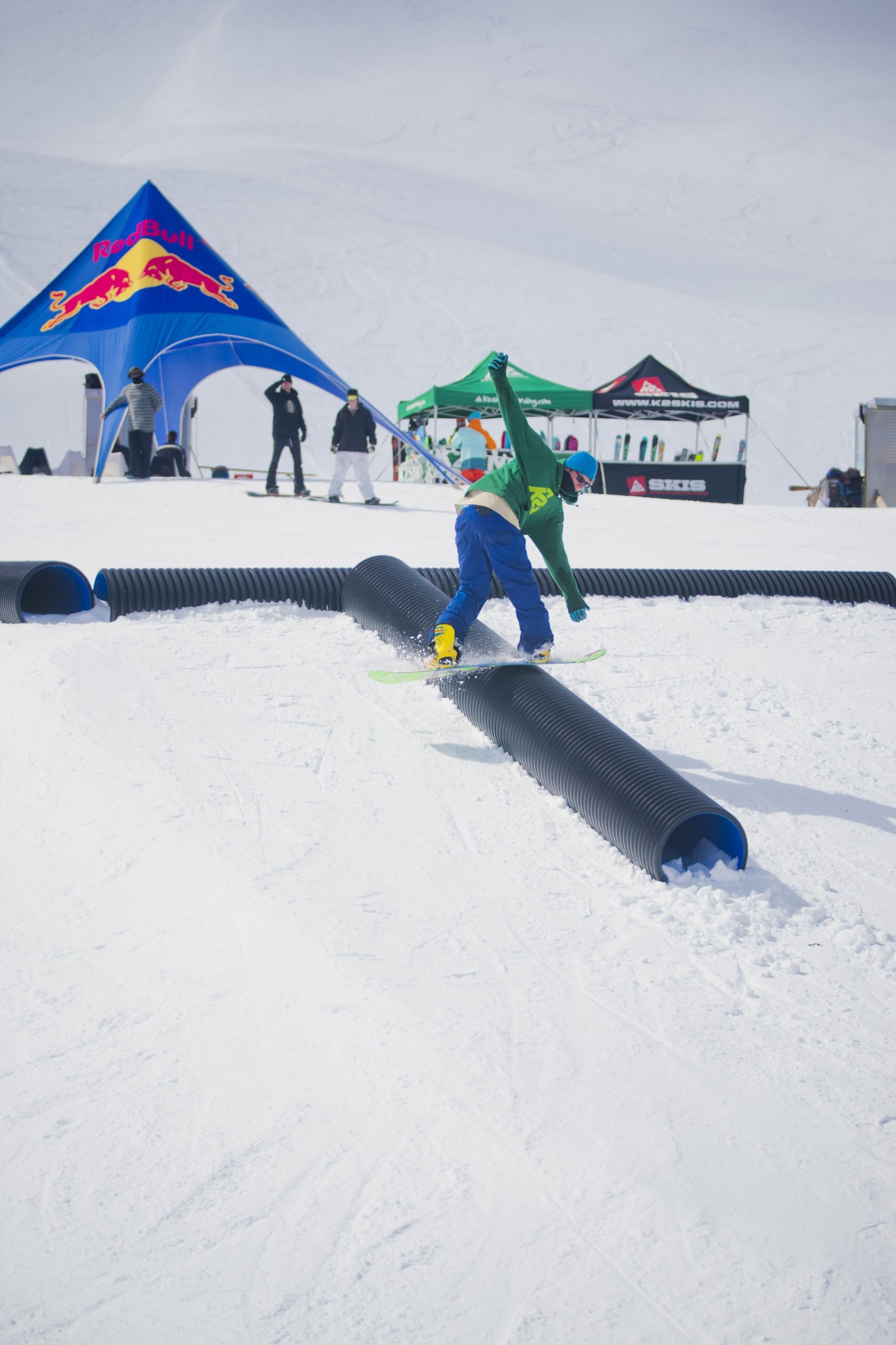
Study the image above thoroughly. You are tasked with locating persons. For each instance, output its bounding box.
[328,389,380,504]
[100,366,163,478]
[451,411,496,484]
[156,431,191,477]
[448,418,466,446]
[264,374,311,495]
[428,352,598,669]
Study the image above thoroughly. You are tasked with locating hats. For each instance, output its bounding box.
[281,374,292,384]
[347,389,358,396]
[564,451,597,480]
[128,366,144,377]
[468,411,481,421]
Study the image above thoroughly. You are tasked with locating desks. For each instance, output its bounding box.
[396,445,589,493]
[590,460,748,507]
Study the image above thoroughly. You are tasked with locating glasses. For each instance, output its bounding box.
[575,471,590,488]
[347,394,357,400]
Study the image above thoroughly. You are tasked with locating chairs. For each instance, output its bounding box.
[0,443,22,477]
[149,443,187,480]
[102,450,130,479]
[54,449,91,478]
[19,447,54,476]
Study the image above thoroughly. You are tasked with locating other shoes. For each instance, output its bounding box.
[295,489,310,494]
[428,624,457,670]
[329,496,339,503]
[366,498,380,503]
[526,648,550,663]
[267,488,278,494]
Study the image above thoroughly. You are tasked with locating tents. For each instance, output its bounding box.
[0,180,473,484]
[551,355,749,494]
[397,351,593,493]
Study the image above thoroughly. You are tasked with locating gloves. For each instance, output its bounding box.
[570,606,590,622]
[301,431,306,442]
[368,444,375,453]
[488,352,509,370]
[330,445,337,454]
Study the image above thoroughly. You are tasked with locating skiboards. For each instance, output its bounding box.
[789,485,816,491]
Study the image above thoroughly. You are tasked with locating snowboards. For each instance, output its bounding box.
[367,646,606,685]
[564,435,578,451]
[540,430,547,444]
[551,436,561,450]
[639,436,648,460]
[623,432,631,460]
[712,436,722,461]
[658,440,665,461]
[673,451,681,461]
[307,498,400,506]
[614,434,622,459]
[736,440,745,462]
[695,450,704,461]
[501,430,511,449]
[392,425,447,481]
[679,446,688,461]
[246,491,329,498]
[651,434,659,461]
[687,453,696,461]
